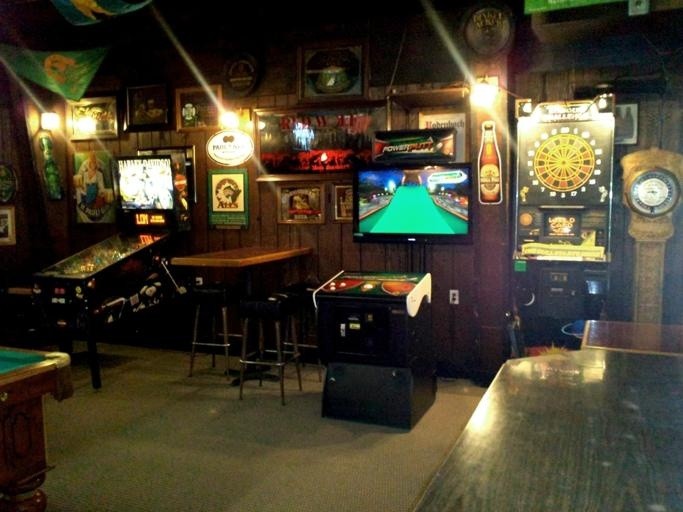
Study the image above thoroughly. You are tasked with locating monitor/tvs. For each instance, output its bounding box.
[353,163,472,246]
[115,156,176,225]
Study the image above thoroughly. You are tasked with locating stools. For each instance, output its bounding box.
[239,294,302,407]
[282,284,329,382]
[189,285,246,381]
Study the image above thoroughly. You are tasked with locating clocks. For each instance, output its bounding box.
[622,147,683,324]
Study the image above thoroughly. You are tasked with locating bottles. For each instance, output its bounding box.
[479,121,502,203]
[39,137,64,200]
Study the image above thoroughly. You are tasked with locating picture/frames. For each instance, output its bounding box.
[276,183,324,223]
[0,163,17,203]
[333,184,354,221]
[63,97,116,135]
[128,85,172,131]
[0,206,15,245]
[72,149,118,226]
[176,85,221,131]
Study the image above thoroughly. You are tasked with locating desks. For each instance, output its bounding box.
[172,245,310,375]
